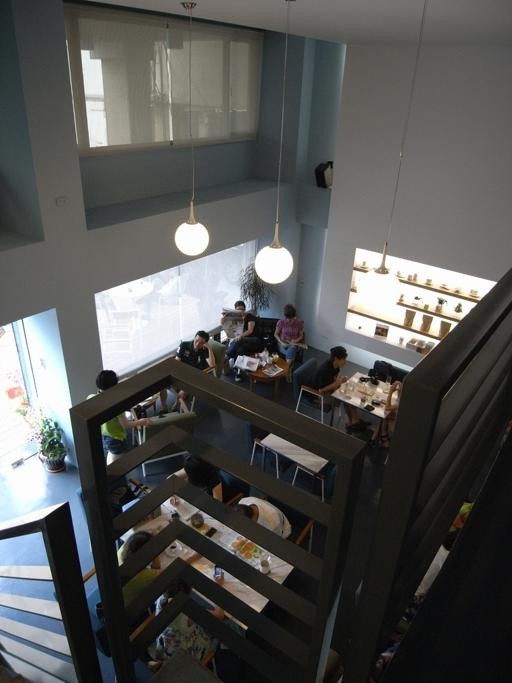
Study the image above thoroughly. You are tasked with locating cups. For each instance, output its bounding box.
[259,560,270,574]
[168,542,177,552]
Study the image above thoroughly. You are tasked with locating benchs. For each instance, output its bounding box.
[244,318,304,365]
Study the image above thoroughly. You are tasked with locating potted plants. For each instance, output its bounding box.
[17,403,70,471]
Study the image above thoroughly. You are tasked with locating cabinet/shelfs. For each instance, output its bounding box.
[345,247,498,356]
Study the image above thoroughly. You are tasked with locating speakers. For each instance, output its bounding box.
[313,162,333,189]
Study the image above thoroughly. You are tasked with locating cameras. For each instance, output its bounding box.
[366,368,379,384]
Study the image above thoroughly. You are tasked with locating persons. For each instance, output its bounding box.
[87,300,478,667]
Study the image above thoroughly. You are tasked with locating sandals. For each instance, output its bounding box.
[365,439,377,448]
[378,435,388,445]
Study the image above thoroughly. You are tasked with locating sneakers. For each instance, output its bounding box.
[235,374,242,382]
[350,417,372,431]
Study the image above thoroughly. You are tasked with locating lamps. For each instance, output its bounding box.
[356,0,428,309]
[253,1,295,286]
[174,1,210,257]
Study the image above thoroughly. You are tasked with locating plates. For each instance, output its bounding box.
[165,541,183,556]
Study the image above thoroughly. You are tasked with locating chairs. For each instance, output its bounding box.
[246,422,336,504]
[135,411,196,477]
[53,566,228,683]
[168,337,226,406]
[220,470,313,553]
[293,357,331,424]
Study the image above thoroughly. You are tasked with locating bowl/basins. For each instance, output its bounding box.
[191,514,204,528]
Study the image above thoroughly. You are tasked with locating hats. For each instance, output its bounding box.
[330,345,348,360]
